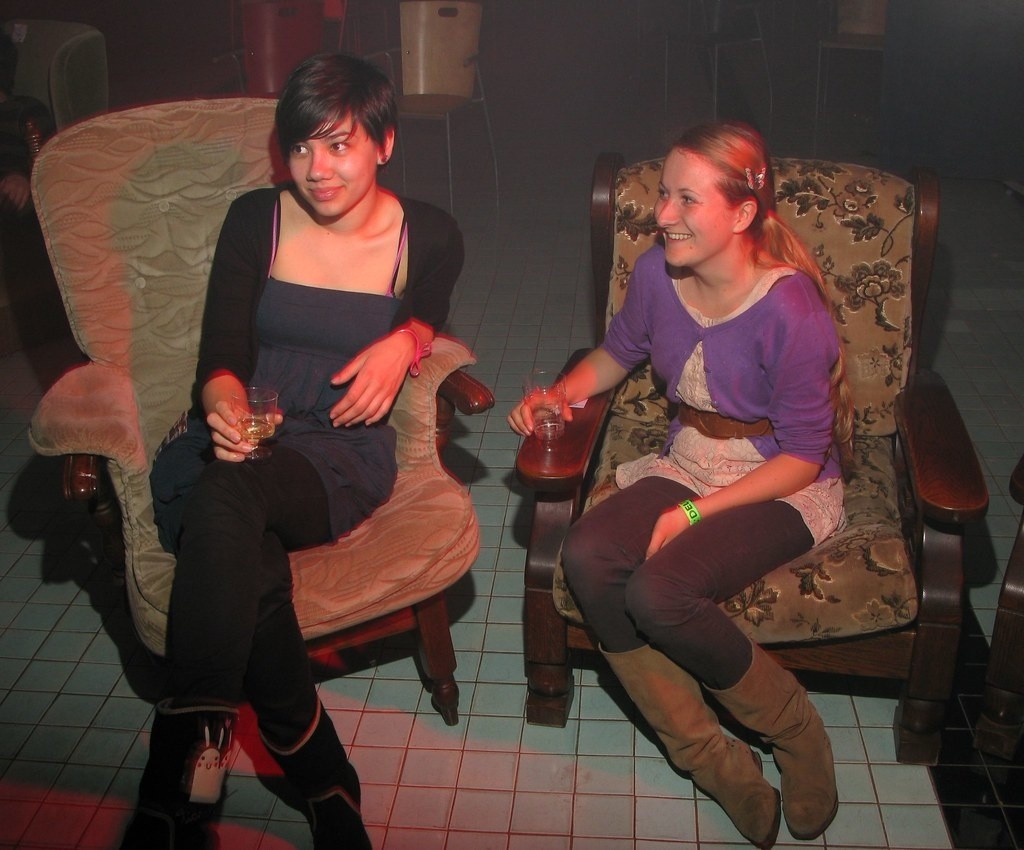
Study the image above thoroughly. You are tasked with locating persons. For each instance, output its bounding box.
[0,35,59,223]
[507,118,856,848]
[118,52,465,849]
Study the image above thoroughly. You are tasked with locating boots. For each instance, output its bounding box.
[599,644,781,850]
[118,698,239,850]
[258,694,372,850]
[702,639,838,840]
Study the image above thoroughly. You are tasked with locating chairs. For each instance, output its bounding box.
[27,96,495,727]
[229,0,390,100]
[393,0,499,214]
[512,150,991,767]
[634,0,779,135]
[968,453,1023,784]
[810,0,890,147]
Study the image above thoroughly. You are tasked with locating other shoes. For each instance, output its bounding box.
[25,340,77,384]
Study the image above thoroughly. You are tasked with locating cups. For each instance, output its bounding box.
[522,369,566,443]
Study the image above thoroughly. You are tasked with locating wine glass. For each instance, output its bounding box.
[232,387,279,460]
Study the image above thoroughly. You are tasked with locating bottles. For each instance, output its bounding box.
[23,119,43,155]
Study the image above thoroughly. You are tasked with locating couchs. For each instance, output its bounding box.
[0,18,111,359]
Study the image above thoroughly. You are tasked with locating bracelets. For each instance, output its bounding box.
[678,499,701,526]
[397,328,432,377]
